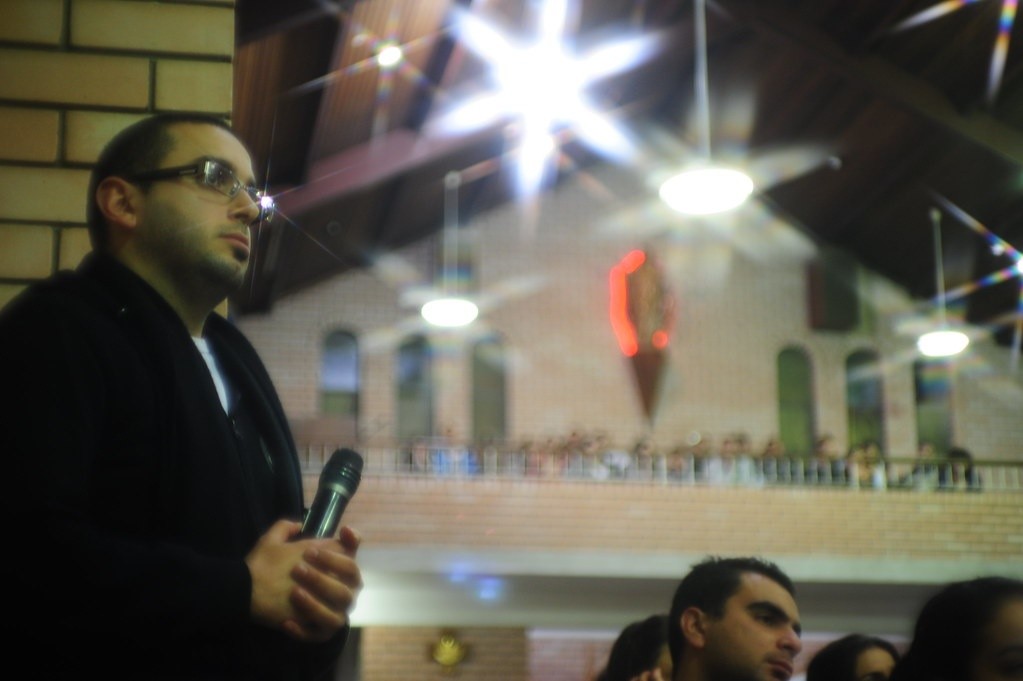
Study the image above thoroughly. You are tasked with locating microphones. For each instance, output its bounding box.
[295,448,364,543]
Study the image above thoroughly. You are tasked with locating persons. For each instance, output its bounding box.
[797,437,983,490]
[807,633,899,681]
[405,425,796,486]
[889,576,1023,681]
[0,111,362,681]
[592,615,673,681]
[667,557,802,681]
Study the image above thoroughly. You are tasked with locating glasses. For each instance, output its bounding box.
[126,160,276,223]
